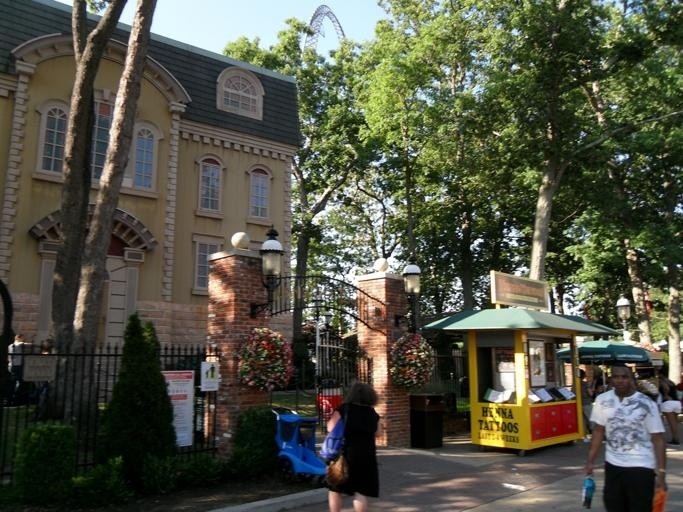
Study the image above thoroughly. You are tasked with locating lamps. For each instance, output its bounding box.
[311,293,323,322]
[374,258,388,272]
[393,252,422,333]
[230,231,249,248]
[250,224,285,318]
[324,307,332,331]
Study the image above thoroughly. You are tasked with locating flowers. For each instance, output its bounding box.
[389,333,435,389]
[235,327,293,393]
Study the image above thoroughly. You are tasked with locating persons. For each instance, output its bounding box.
[570,368,597,433]
[318,379,381,512]
[582,363,666,512]
[7,334,48,398]
[657,362,682,445]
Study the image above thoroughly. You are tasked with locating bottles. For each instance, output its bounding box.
[579,475,595,508]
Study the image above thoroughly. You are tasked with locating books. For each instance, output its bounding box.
[546,385,566,401]
[534,386,553,401]
[527,387,541,403]
[482,387,512,403]
[557,386,577,400]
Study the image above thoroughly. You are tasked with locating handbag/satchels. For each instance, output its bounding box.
[320,419,345,461]
[325,455,348,486]
